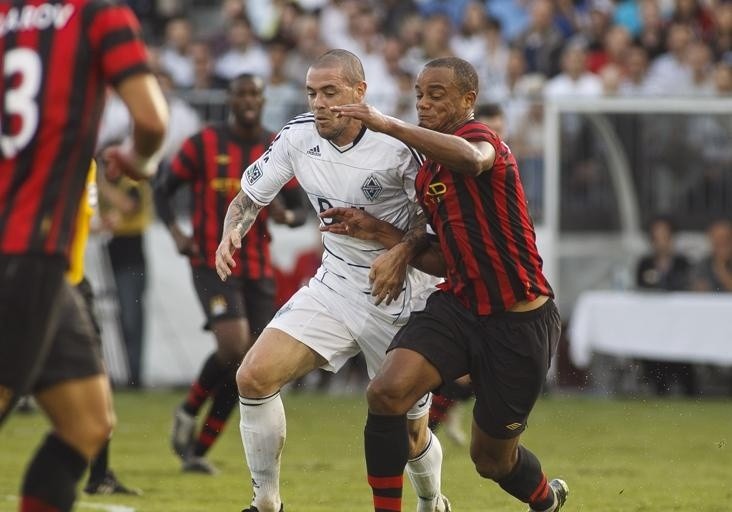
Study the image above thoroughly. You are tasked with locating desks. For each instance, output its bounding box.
[567,291,732,369]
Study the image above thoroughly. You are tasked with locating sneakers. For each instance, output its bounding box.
[527,479,569,512]
[435,493,451,511]
[173,407,196,458]
[183,457,220,474]
[84,471,143,497]
[242,503,284,512]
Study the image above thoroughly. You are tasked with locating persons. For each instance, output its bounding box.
[215,50,450,510]
[315,57,569,510]
[64,157,138,492]
[152,74,304,467]
[0,0,731,394]
[1,0,167,511]
